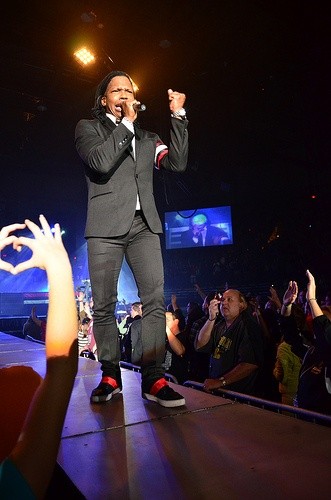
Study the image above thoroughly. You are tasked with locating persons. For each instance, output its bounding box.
[162,284,281,410]
[74,71,189,407]
[23,283,171,372]
[181,210,228,246]
[265,269,331,426]
[0,214,79,500]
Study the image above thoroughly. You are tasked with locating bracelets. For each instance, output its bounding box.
[307,298,317,303]
[209,317,217,321]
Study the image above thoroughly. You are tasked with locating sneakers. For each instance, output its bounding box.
[90,374,123,403]
[140,378,185,407]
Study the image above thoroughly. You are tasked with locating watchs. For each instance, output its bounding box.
[219,376,226,386]
[174,109,185,116]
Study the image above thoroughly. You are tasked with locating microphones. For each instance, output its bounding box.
[133,102,147,112]
